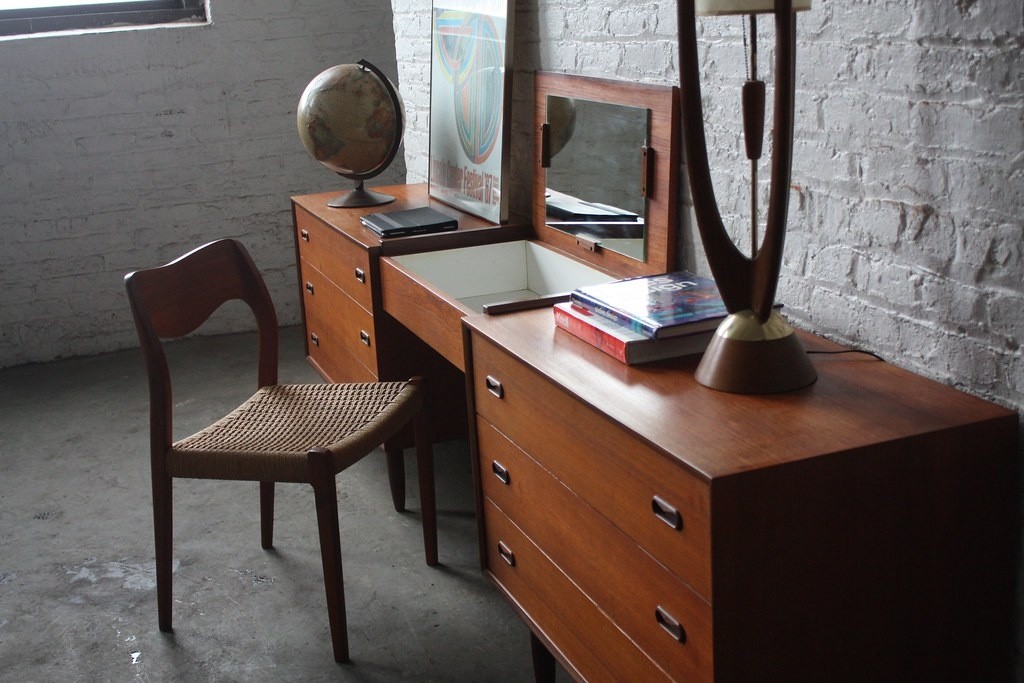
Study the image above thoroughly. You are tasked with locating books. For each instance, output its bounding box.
[545,202,638,222]
[571,271,784,341]
[360,207,458,238]
[554,302,717,367]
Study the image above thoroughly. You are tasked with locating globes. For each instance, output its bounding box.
[298,58,407,208]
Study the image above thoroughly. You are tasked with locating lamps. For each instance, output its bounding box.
[677,0,819,396]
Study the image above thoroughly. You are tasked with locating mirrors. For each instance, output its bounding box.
[545,94,650,263]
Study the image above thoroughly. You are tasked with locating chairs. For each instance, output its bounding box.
[124,237,438,664]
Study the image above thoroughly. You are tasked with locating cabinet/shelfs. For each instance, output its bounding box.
[460,292,1020,683]
[290,182,530,451]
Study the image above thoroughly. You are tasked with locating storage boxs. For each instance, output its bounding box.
[384,74,679,373]
